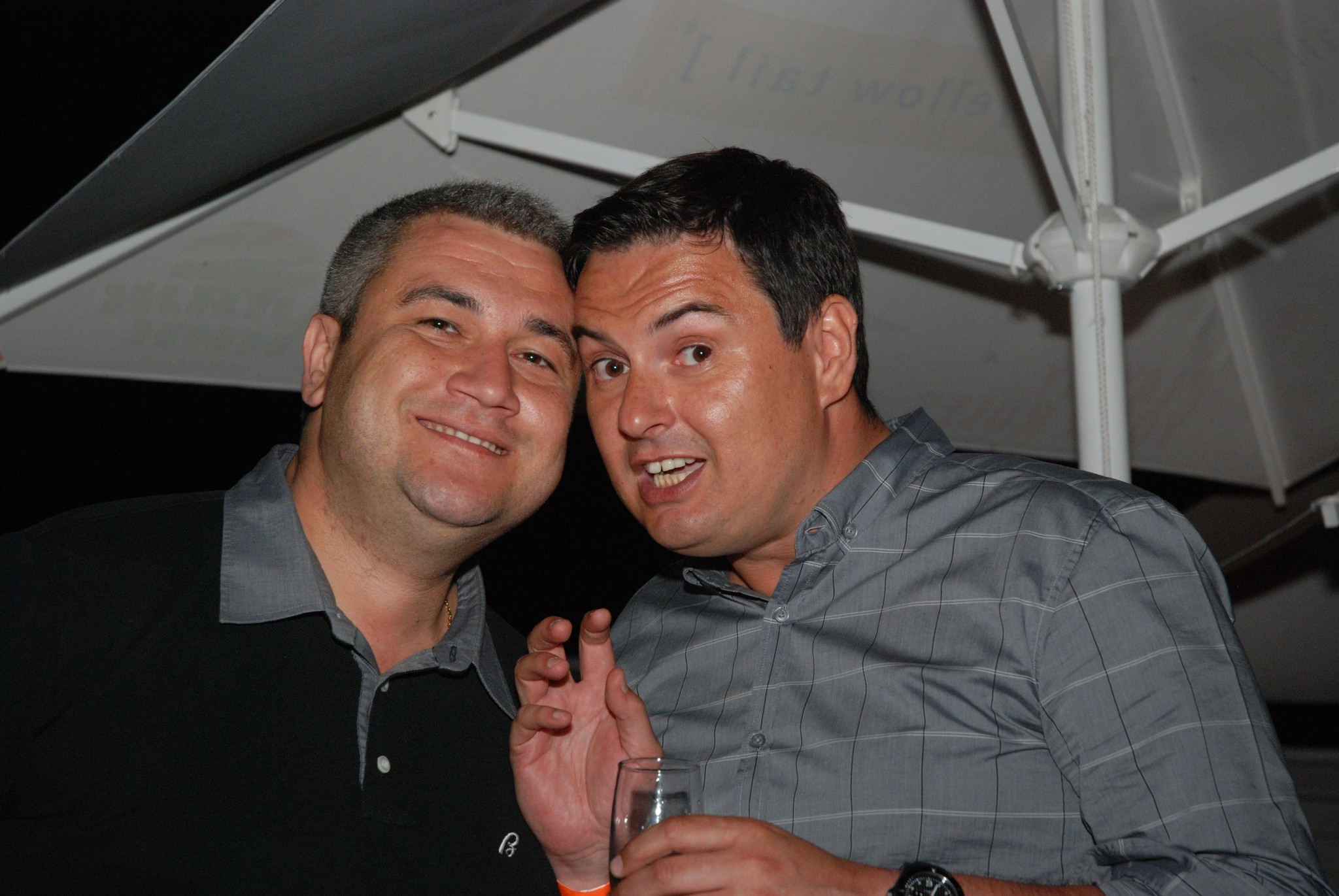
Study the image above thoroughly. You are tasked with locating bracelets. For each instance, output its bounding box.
[556,879,611,896]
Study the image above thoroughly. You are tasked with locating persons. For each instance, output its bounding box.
[509,145,1339,896]
[0,186,582,896]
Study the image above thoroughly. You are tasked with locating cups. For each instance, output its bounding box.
[608,757,705,890]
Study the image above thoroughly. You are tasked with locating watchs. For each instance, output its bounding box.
[881,859,965,896]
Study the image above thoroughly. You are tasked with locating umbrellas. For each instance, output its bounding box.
[0,0,1339,501]
